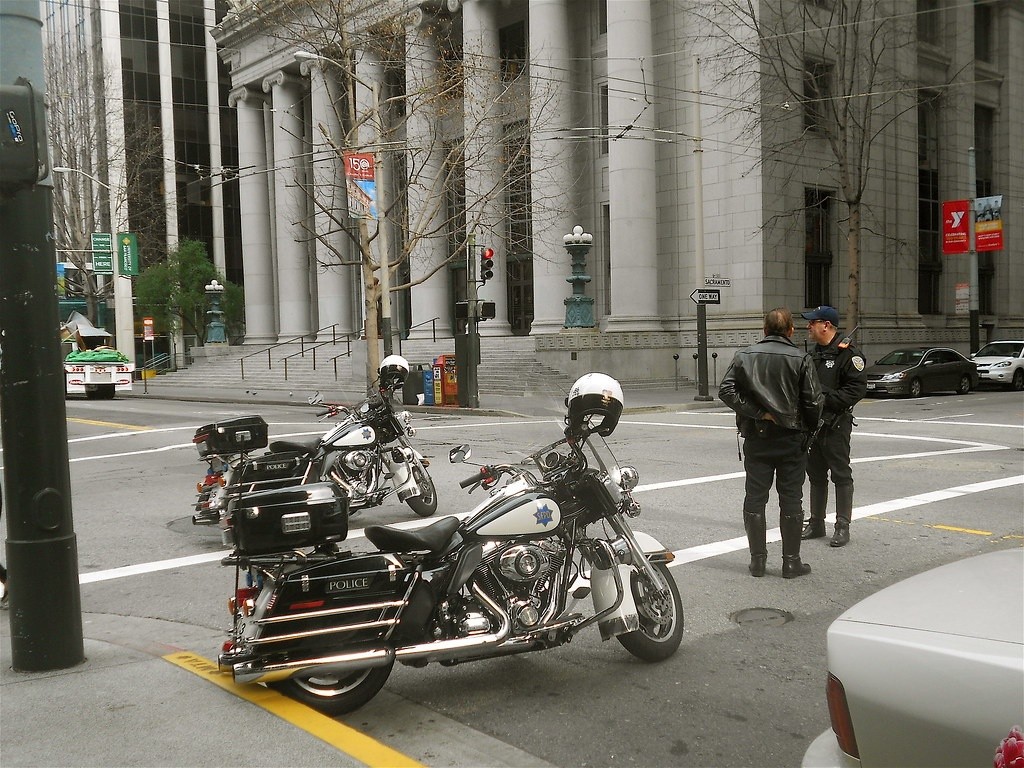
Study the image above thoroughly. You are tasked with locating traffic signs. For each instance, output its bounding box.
[689,288,721,305]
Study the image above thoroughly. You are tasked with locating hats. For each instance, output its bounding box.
[801,306,839,329]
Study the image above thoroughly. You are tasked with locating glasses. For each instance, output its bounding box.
[808,320,825,328]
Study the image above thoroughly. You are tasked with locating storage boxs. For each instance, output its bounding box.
[193,415,270,456]
[224,480,352,552]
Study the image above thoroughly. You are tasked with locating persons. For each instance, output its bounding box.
[976,199,1001,222]
[718,307,825,580]
[801,305,867,547]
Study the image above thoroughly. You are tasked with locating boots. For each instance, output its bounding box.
[801,480,829,540]
[780,508,811,578]
[743,509,768,577]
[830,482,855,545]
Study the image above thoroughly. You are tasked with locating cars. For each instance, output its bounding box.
[867,345,981,398]
[968,342,1024,392]
[800,544,1024,768]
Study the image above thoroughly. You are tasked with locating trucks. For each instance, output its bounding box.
[60,308,137,401]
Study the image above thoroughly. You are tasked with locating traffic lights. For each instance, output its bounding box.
[476,301,496,318]
[0,76,48,194]
[480,247,495,281]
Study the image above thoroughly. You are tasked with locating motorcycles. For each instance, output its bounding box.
[218,413,683,717]
[191,365,440,547]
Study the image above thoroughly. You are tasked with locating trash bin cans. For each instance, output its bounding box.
[402,362,432,405]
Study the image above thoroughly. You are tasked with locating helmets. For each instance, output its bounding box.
[568,372,624,437]
[380,354,410,389]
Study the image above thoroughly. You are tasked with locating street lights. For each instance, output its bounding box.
[294,51,396,399]
[53,165,117,322]
